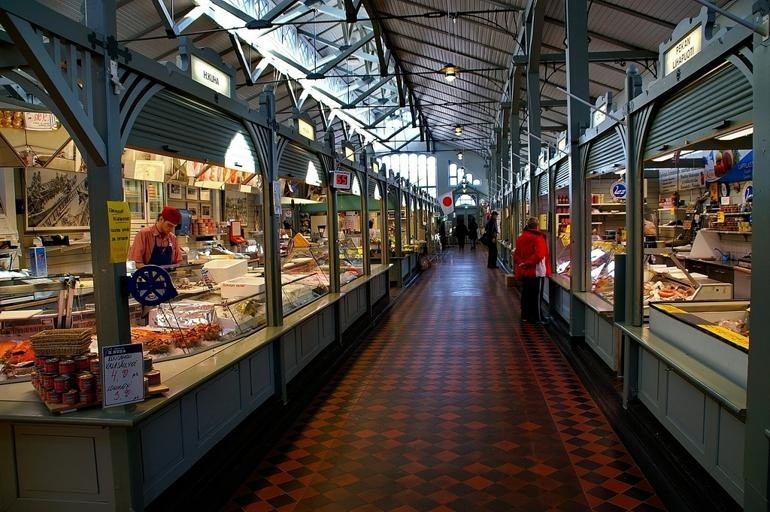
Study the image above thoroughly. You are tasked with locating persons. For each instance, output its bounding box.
[513,216,554,325]
[369,219,376,239]
[456,219,468,255]
[643,212,657,235]
[128,205,184,271]
[279,221,291,239]
[485,211,500,269]
[469,216,478,250]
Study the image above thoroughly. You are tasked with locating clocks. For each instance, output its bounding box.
[720,183,730,197]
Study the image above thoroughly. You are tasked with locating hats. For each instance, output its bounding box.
[490,211,499,215]
[161,205,182,225]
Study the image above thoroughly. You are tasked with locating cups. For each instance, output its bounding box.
[722,255,729,260]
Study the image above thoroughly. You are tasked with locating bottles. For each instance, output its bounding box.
[557,194,570,232]
[658,191,678,209]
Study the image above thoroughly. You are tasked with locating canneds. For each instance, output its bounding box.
[709,215,752,231]
[29,353,162,408]
[721,202,753,213]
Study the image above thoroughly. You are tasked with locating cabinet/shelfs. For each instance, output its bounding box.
[252,230,292,256]
[556,198,626,245]
[122,178,164,247]
[643,208,752,299]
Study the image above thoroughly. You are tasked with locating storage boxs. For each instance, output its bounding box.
[203,259,248,283]
[221,277,266,300]
[209,255,234,259]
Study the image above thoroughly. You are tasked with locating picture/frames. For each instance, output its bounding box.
[169,183,212,220]
[222,191,248,227]
[23,167,90,235]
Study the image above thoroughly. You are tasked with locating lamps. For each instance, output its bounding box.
[444,63,456,82]
[289,184,296,192]
[455,125,462,136]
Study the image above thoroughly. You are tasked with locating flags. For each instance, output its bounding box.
[437,191,455,217]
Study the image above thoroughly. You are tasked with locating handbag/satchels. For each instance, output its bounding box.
[481,233,489,246]
